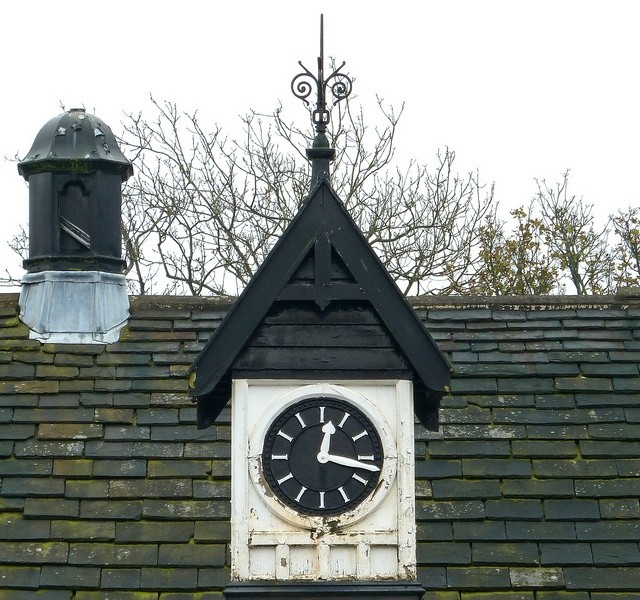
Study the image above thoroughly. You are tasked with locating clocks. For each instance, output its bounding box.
[263,396,383,515]
